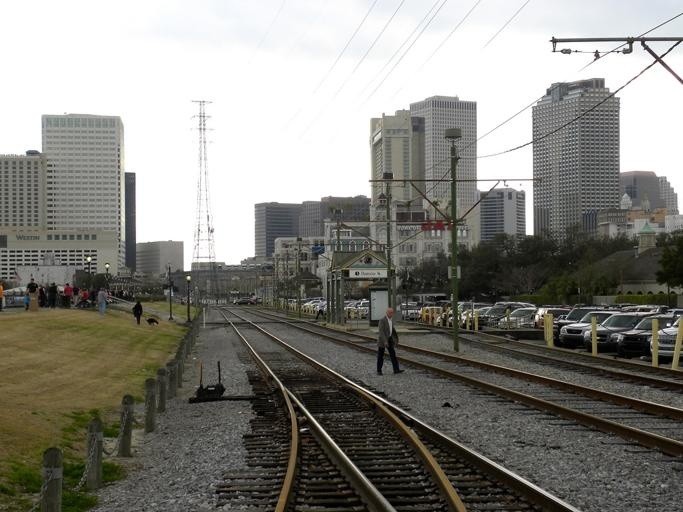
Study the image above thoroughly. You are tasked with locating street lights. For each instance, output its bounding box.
[383,172,395,309]
[334,208,343,251]
[443,127,464,354]
[186,275,192,321]
[86,255,111,279]
[296,236,303,272]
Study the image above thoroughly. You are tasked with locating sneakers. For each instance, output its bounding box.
[377,369,383,376]
[393,370,405,374]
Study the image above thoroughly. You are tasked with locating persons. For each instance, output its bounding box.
[0,281,4,312]
[376,308,404,375]
[132,301,143,326]
[21,274,135,316]
[314,297,327,320]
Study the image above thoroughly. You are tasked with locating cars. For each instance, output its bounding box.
[233,296,256,305]
